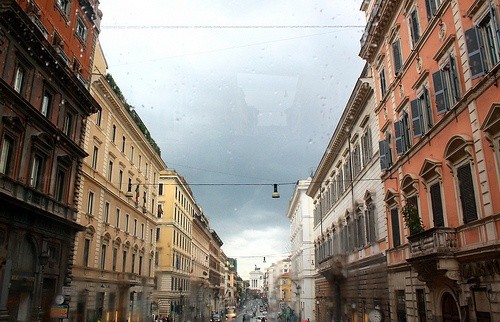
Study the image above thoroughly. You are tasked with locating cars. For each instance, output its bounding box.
[209,295,269,322]
